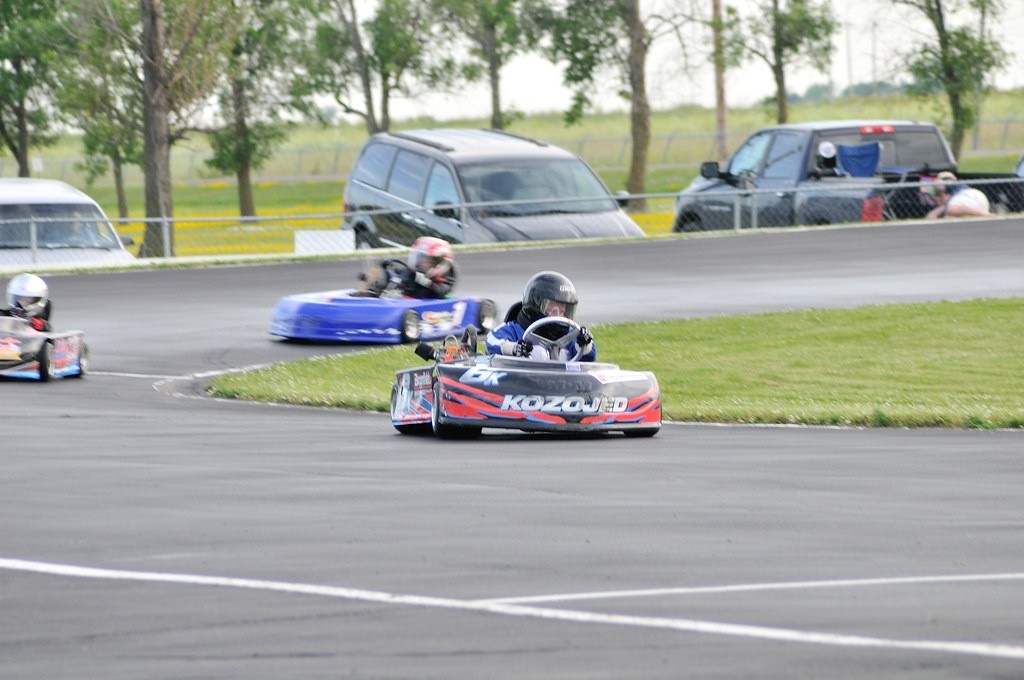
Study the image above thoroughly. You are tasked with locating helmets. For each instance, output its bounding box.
[521,270,578,323]
[409,236,453,257]
[6,273,50,316]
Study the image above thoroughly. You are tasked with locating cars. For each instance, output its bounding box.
[0,179,137,269]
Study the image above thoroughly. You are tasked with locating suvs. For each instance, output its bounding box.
[341,126,649,239]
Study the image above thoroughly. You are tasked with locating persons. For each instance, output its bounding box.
[0,273,53,332]
[806,141,994,222]
[485,271,597,361]
[380,235,458,302]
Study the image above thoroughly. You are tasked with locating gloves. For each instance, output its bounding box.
[392,265,416,279]
[511,338,533,358]
[576,326,594,348]
[31,317,51,332]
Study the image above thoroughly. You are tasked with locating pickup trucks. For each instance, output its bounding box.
[671,120,1024,232]
